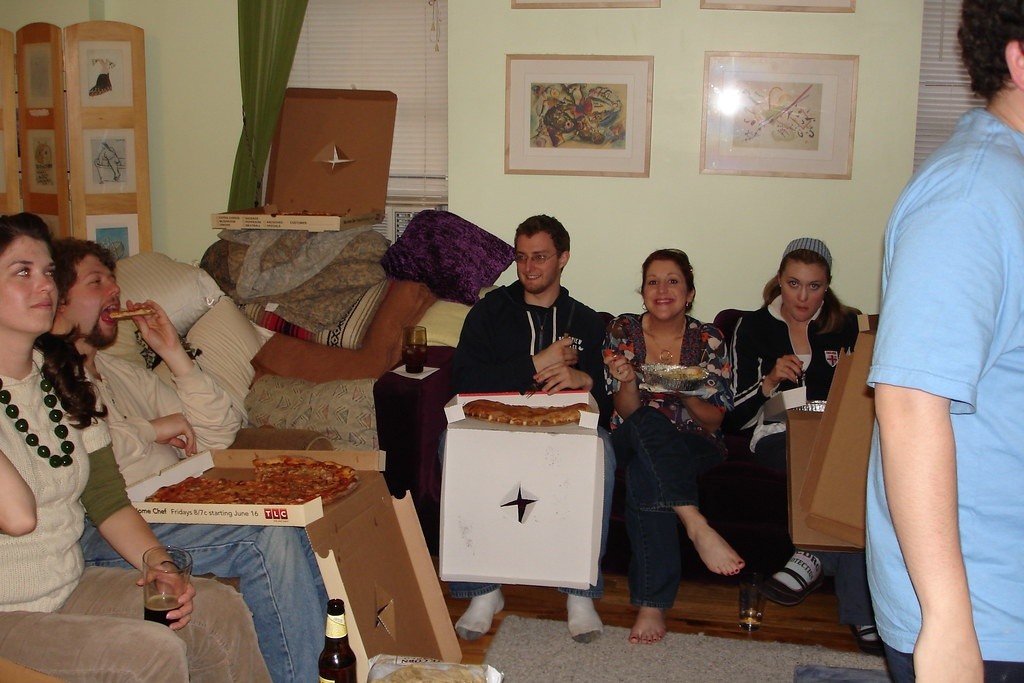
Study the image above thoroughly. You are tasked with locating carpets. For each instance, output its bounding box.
[480,614,886,683]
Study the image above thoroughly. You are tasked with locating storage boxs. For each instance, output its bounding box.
[797,313,878,550]
[125,447,463,683]
[764,386,807,421]
[788,409,862,551]
[438,390,606,591]
[212,87,398,233]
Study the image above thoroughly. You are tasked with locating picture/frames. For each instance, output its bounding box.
[511,0,662,10]
[699,0,857,13]
[699,50,860,181]
[504,53,654,179]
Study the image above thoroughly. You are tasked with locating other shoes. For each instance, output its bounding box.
[756,567,824,605]
[850,622,884,655]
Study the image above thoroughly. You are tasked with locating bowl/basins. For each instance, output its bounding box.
[642,366,709,390]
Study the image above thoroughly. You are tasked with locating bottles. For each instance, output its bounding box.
[318,597,358,683]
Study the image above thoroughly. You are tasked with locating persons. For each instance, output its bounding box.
[723,238,881,654]
[53,237,330,683]
[865,0,1024,683]
[449,212,616,642]
[602,248,744,644]
[0,212,272,683]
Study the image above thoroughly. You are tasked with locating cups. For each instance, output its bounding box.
[142,546,192,624]
[403,325,427,373]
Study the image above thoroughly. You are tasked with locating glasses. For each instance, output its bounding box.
[515,251,561,264]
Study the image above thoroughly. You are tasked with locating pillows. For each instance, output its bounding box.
[155,294,264,426]
[114,250,226,337]
[379,209,515,307]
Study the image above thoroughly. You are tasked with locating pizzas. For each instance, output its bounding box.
[109,307,155,321]
[145,454,360,507]
[462,399,590,427]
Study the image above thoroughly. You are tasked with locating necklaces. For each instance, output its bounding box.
[659,349,673,364]
[0,378,75,468]
